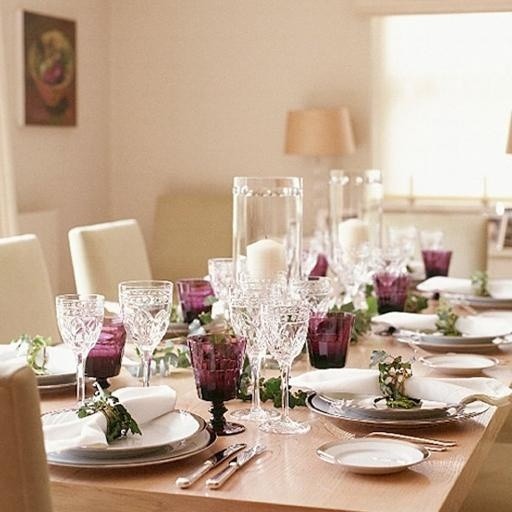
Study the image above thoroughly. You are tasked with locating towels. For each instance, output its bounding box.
[288,368,479,405]
[371,311,440,330]
[81,385,176,449]
[417,276,480,294]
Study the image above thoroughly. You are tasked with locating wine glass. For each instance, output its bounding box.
[56,227,457,436]
[55,293,105,401]
[229,270,282,420]
[256,307,309,434]
[118,279,175,386]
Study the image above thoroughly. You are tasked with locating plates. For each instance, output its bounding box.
[393,274,512,372]
[0,347,91,396]
[305,374,493,427]
[314,436,434,477]
[25,403,218,467]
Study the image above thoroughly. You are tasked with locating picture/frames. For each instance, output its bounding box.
[16,9,78,130]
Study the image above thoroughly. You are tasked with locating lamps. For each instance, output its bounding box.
[284,106,357,212]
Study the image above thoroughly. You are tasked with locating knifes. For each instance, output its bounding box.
[174,443,268,491]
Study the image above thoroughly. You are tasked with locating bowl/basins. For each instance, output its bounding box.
[29,29,75,109]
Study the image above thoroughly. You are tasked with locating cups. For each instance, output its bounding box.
[310,312,357,369]
[329,171,384,270]
[228,175,305,318]
[419,249,453,279]
[373,272,411,313]
[188,332,244,436]
[86,316,126,388]
[176,277,215,322]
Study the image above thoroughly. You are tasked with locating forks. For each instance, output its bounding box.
[317,416,457,449]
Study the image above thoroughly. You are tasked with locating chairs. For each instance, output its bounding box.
[69,219,153,304]
[0,233,63,346]
